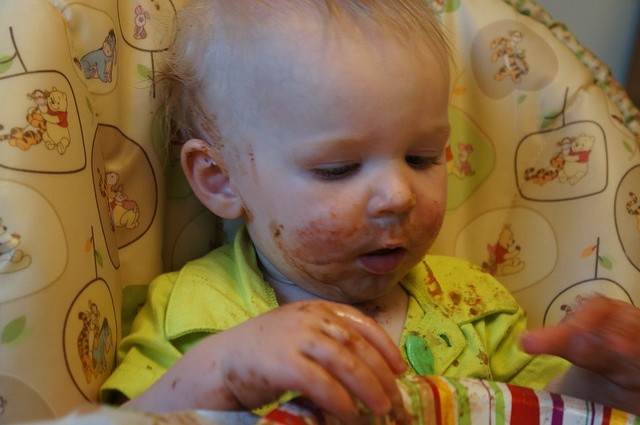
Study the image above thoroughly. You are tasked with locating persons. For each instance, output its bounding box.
[99,0,640,424]
